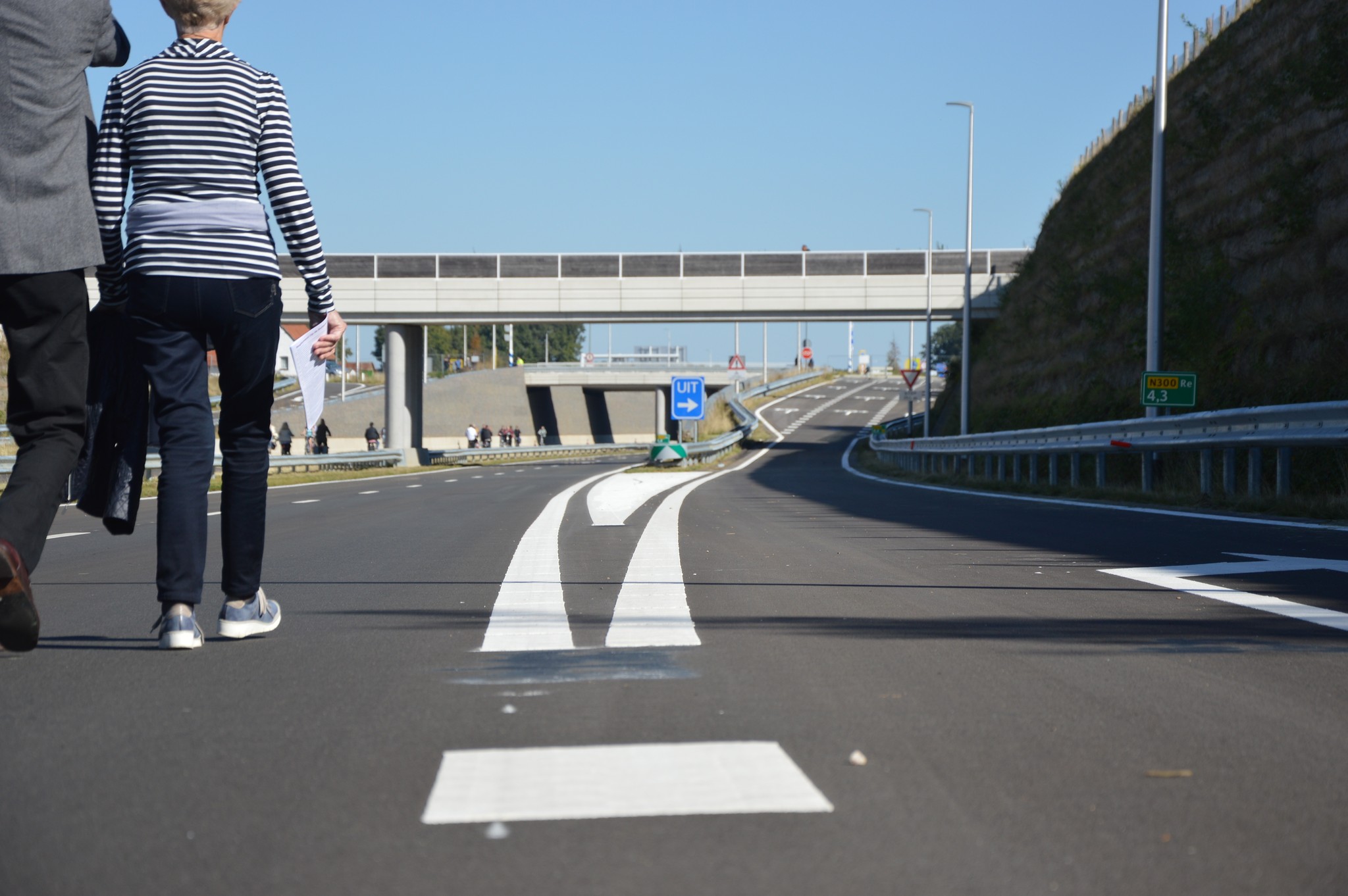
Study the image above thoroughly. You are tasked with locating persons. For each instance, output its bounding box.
[268,355,546,456]
[91,0,346,647]
[0,0,131,648]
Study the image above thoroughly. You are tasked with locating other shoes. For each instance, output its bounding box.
[1,538,39,654]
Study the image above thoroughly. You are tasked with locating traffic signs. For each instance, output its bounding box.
[670,376,704,420]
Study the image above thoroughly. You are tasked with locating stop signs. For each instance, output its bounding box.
[802,348,812,359]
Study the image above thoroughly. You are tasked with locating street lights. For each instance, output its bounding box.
[945,98,974,435]
[913,208,934,441]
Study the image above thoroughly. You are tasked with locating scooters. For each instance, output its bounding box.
[472,433,545,449]
[367,439,378,451]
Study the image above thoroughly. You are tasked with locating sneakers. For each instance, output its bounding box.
[150,608,204,649]
[217,586,280,639]
